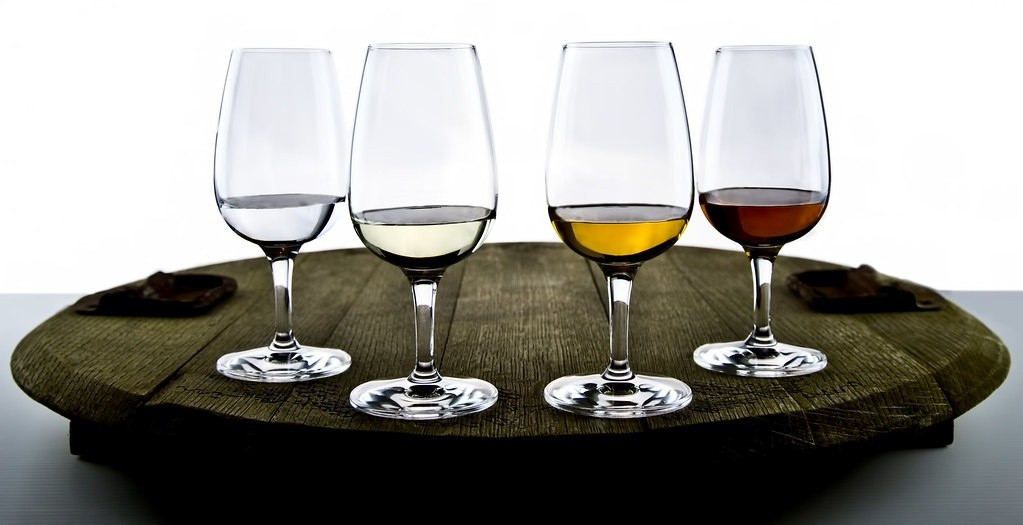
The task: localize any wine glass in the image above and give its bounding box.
[697,44,833,379]
[346,43,499,422]
[542,38,695,421]
[214,46,352,383]
